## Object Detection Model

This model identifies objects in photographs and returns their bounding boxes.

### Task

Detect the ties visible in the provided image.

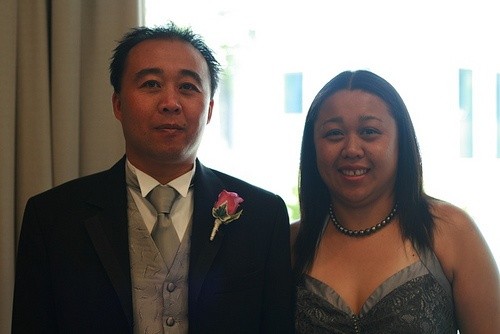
[144,185,181,272]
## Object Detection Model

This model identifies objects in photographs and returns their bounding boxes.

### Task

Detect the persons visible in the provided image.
[290,70,500,334]
[11,26,290,334]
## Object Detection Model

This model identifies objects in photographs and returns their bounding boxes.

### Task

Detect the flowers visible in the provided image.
[209,190,244,241]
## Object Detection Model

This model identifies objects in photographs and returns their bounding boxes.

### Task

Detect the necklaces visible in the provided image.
[329,200,399,237]
